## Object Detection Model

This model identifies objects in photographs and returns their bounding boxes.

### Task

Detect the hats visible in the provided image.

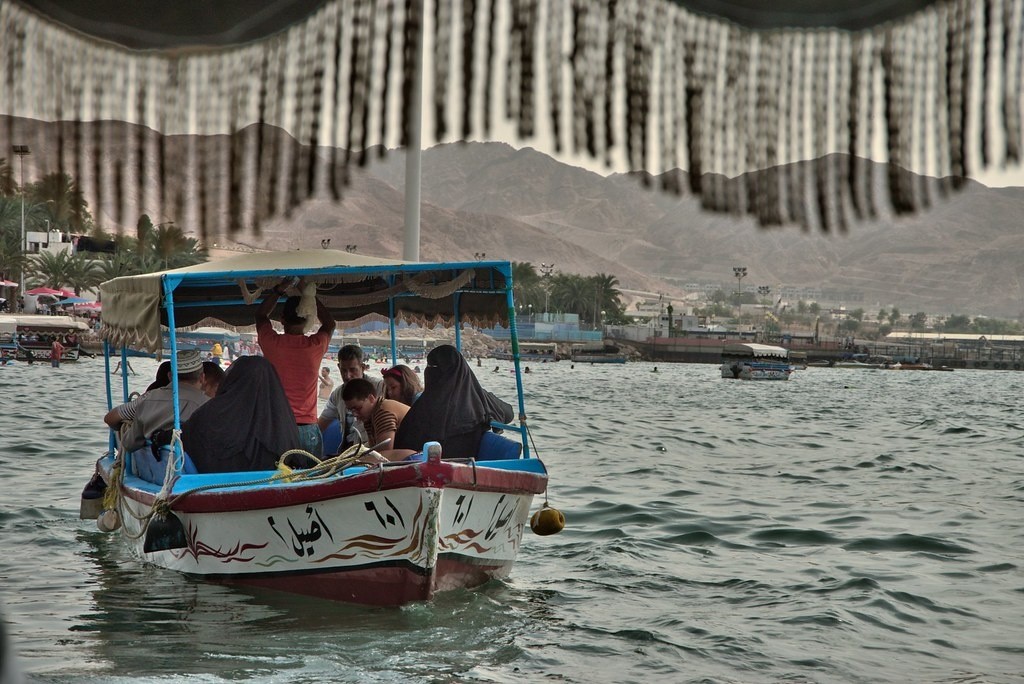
[177,348,203,373]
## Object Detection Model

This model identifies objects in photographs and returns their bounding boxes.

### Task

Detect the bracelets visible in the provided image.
[273,285,284,296]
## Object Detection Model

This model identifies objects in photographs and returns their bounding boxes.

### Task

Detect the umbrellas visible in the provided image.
[0,280,101,321]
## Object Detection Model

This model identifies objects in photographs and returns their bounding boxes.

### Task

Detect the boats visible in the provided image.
[80,246,549,614]
[788,351,808,371]
[321,335,433,360]
[81,340,127,357]
[175,327,240,365]
[571,343,626,364]
[721,343,793,381]
[491,341,558,361]
[16,321,89,362]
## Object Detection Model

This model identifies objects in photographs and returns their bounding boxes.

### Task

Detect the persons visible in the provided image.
[3,300,9,311]
[17,297,24,311]
[362,351,421,373]
[50,336,64,367]
[181,356,305,474]
[201,338,262,365]
[380,345,514,462]
[463,348,469,358]
[527,349,554,362]
[342,378,411,450]
[0,344,37,365]
[255,275,336,464]
[112,360,136,376]
[477,356,481,365]
[494,367,499,372]
[319,345,388,452]
[383,364,424,407]
[51,304,56,316]
[319,367,334,399]
[105,350,226,431]
[524,367,531,372]
